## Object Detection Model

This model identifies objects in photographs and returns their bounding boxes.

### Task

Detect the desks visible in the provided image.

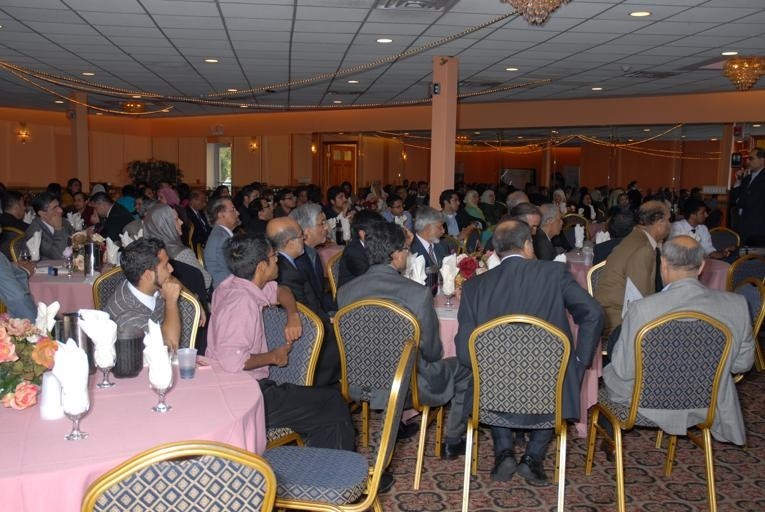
[11,255,114,316]
[8,338,268,509]
[424,286,614,426]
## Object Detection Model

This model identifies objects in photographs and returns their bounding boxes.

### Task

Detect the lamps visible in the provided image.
[723,56,765,91]
[122,102,144,113]
[501,0,570,24]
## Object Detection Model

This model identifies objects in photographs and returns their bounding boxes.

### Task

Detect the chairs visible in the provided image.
[591,311,730,512]
[139,280,208,355]
[333,306,450,454]
[270,334,422,511]
[453,309,572,512]
[250,305,321,443]
[81,436,276,512]
[92,273,149,310]
[10,202,756,260]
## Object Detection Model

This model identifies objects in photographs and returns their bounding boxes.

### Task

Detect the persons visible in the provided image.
[438,178,735,333]
[102,236,184,351]
[205,181,450,387]
[0,177,211,324]
[729,146,765,245]
[205,232,395,493]
[336,222,472,460]
[601,236,756,460]
[454,221,606,486]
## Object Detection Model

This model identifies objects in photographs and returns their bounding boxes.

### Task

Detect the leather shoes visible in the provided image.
[396,421,419,442]
[441,438,465,460]
[601,439,616,462]
[378,474,395,493]
[490,450,517,482]
[516,455,549,487]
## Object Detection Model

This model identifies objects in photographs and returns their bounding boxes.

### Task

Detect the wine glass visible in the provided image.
[441,287,456,306]
[575,242,583,256]
[61,256,74,277]
[60,386,91,440]
[146,365,175,413]
[93,343,117,389]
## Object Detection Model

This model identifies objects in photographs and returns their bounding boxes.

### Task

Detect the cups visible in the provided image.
[113,326,146,379]
[176,348,198,380]
[62,310,96,375]
[18,249,32,262]
[426,272,439,297]
[83,242,100,278]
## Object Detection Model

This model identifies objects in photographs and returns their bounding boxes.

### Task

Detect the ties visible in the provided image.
[428,244,437,264]
[656,247,663,293]
[197,212,205,247]
